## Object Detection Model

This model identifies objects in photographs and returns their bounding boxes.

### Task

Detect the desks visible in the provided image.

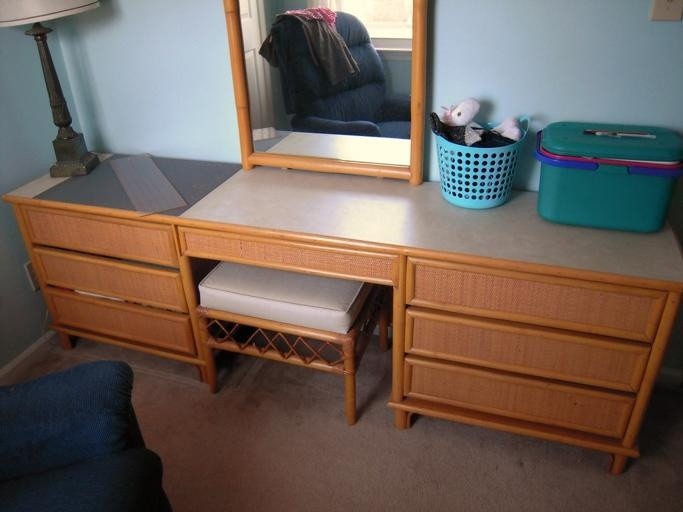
[2,151,682,476]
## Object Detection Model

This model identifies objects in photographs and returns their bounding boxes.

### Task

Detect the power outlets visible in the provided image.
[650,0,682,21]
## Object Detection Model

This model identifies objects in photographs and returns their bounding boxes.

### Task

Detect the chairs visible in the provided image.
[274,7,410,140]
[0,361,173,511]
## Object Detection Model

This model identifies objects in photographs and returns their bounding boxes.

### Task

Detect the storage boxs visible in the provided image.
[531,121,683,234]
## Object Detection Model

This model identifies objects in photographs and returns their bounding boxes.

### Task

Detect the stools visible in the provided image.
[196,262,388,426]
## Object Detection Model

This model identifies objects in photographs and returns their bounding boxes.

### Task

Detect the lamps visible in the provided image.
[1,1,102,178]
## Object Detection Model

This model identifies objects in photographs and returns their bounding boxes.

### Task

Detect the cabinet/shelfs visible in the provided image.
[397,255,681,477]
[4,197,205,392]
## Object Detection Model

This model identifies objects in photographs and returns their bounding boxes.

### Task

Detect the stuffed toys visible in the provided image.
[422,96,522,154]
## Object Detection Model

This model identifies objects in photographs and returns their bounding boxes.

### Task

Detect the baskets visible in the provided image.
[430,116,530,209]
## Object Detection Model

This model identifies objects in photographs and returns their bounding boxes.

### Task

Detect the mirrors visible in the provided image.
[224,1,428,185]
